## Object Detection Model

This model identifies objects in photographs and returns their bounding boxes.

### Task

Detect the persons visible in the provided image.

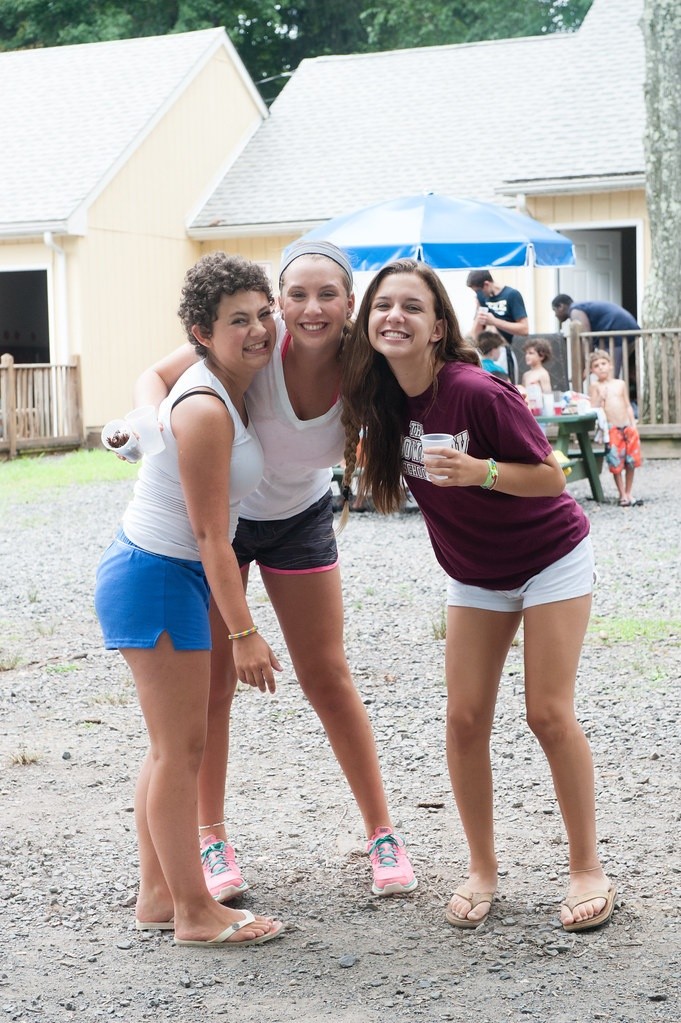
[337,257,618,927]
[521,338,552,394]
[552,294,640,379]
[116,240,418,900]
[467,270,530,345]
[95,252,285,945]
[477,331,506,373]
[588,350,643,507]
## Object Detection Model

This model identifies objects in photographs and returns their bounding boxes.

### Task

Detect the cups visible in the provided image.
[478,307,489,315]
[122,404,165,458]
[101,419,145,464]
[420,433,454,482]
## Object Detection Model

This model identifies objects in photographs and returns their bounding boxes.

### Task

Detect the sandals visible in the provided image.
[632,498,644,506]
[618,499,630,506]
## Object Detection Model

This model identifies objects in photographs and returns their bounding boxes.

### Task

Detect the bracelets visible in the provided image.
[480,458,498,491]
[228,626,258,640]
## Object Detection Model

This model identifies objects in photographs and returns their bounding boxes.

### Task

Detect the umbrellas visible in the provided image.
[282,192,577,274]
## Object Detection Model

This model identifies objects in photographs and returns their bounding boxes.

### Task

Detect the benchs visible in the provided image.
[544,446,618,501]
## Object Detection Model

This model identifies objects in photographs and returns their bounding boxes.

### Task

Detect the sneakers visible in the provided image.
[365,826,426,895]
[200,834,251,901]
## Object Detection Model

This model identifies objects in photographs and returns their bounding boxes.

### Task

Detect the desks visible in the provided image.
[535,412,605,502]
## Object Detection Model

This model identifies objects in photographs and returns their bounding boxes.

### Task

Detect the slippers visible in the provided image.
[171,909,283,947]
[562,887,625,930]
[135,914,177,931]
[445,884,498,926]
